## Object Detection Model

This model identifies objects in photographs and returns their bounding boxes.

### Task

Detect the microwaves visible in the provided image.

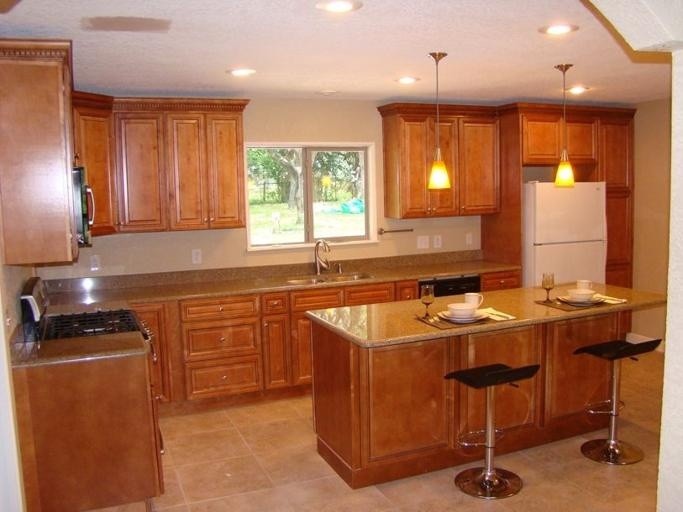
[70,165,96,248]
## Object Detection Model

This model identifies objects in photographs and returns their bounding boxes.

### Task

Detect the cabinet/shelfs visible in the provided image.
[482,269,523,291]
[181,291,292,414]
[379,103,502,219]
[520,110,598,166]
[291,280,420,397]
[600,106,636,288]
[1,38,113,266]
[112,96,250,230]
[134,302,185,417]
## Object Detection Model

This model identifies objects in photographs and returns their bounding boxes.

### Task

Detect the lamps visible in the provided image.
[554,64,576,187]
[427,52,452,189]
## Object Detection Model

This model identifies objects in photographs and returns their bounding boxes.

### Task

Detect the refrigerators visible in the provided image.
[521,180,608,288]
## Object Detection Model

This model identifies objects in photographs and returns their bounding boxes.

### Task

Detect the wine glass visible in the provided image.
[541,272,555,303]
[421,284,435,319]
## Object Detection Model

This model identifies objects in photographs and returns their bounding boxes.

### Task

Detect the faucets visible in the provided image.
[314,237,330,275]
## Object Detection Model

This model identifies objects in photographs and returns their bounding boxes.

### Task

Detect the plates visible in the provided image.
[437,310,490,324]
[557,295,607,307]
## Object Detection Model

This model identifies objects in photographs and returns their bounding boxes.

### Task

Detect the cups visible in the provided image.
[464,293,484,308]
[577,279,592,291]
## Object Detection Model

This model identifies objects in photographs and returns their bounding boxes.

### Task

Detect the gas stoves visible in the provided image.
[20,276,152,346]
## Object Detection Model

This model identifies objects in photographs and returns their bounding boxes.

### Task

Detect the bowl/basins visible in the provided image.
[568,289,595,300]
[447,303,475,316]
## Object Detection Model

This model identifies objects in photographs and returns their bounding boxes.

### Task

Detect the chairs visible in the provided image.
[445,364,540,500]
[573,335,663,464]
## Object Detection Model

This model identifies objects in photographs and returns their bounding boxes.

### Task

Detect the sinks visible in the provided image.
[285,277,333,286]
[328,272,374,283]
